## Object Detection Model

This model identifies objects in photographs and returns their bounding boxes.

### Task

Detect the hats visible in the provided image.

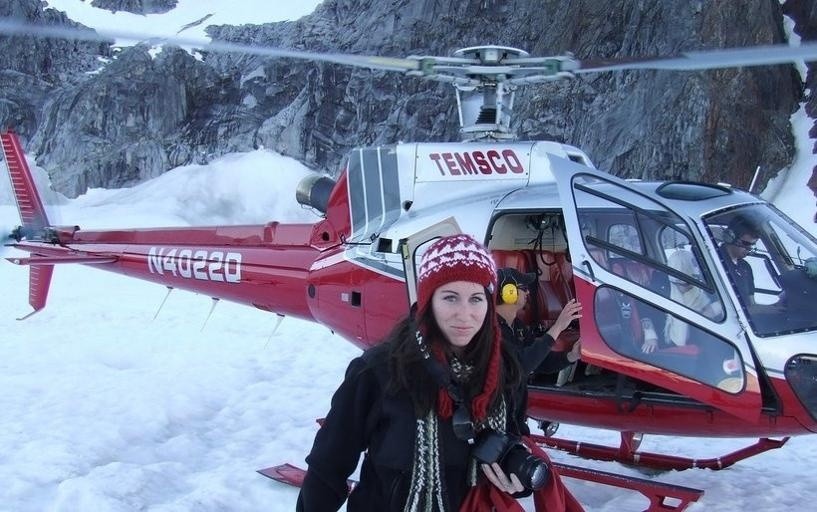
[414,233,499,310]
[497,267,537,302]
[666,248,701,284]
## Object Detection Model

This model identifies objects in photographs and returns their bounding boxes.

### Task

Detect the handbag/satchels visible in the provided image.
[461,436,585,511]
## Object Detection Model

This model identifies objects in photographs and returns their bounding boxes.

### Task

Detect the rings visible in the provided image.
[504,480,511,490]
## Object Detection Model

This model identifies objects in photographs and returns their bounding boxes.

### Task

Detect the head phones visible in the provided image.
[722,226,736,243]
[499,267,518,305]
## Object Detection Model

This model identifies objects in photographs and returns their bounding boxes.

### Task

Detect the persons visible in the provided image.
[720,214,763,306]
[494,267,584,376]
[293,233,533,511]
[634,250,715,354]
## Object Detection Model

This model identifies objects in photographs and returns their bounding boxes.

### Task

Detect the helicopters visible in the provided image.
[0,24,817,512]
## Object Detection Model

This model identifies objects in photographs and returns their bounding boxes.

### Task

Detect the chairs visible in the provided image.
[491,248,701,378]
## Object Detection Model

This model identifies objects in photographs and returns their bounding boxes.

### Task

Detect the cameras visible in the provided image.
[470,428,549,491]
